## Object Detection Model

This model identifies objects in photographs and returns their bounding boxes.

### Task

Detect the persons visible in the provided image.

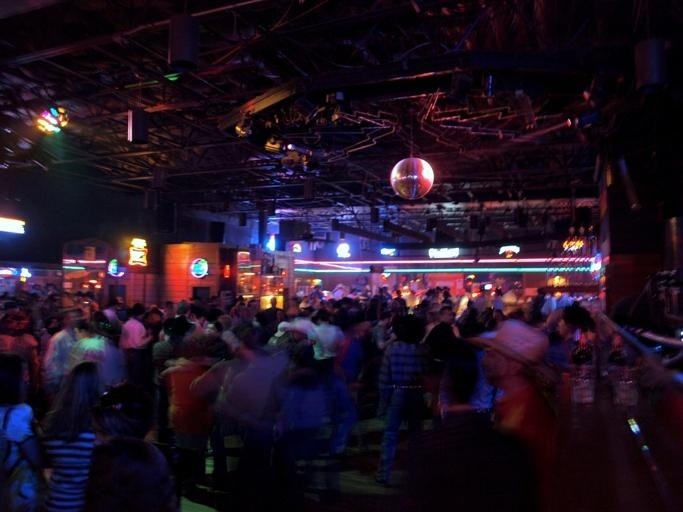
[1,271,681,512]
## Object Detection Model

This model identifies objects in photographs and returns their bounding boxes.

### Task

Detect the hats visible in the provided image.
[464,318,561,385]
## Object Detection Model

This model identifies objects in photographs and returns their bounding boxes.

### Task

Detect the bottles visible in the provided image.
[567,329,640,412]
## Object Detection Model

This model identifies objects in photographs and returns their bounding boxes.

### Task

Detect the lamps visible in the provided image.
[389,115,434,202]
